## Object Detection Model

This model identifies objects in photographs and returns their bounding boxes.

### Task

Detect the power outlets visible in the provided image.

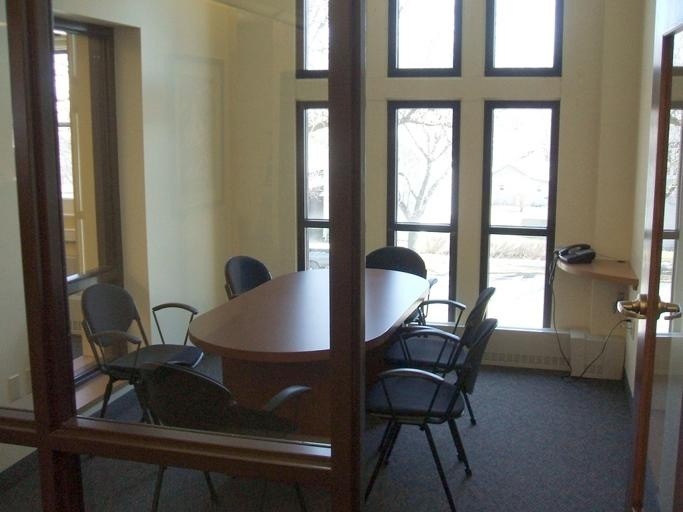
[627,318,636,341]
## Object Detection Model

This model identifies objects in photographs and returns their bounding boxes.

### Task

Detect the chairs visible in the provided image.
[364,317,496,511]
[137,361,312,512]
[80,283,203,423]
[378,288,496,461]
[224,255,273,301]
[365,245,436,324]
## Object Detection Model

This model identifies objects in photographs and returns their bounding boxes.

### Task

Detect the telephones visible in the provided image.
[559,244,596,263]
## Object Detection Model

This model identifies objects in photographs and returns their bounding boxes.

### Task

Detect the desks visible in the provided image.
[186,269,430,490]
